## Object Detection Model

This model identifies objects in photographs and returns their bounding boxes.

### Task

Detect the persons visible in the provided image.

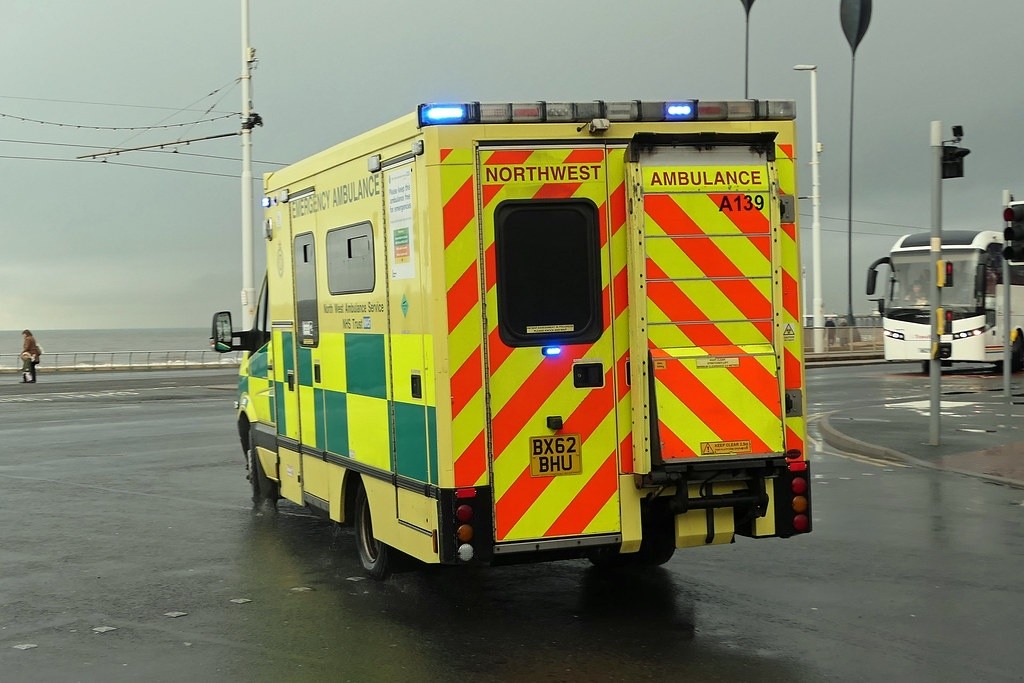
[20,330,40,383]
[903,280,927,301]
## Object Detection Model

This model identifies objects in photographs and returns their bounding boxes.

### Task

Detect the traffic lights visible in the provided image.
[1001,201,1024,265]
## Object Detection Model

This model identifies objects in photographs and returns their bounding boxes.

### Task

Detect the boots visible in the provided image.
[19,372,36,383]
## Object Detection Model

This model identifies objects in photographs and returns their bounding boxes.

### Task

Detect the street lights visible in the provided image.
[793,64,826,352]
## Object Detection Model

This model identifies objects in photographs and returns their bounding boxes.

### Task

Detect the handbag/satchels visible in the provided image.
[36,344,43,355]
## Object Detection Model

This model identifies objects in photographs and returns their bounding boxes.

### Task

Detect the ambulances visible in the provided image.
[210,99,814,579]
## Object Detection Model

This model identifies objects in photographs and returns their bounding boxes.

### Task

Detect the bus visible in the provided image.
[866,227,1024,371]
